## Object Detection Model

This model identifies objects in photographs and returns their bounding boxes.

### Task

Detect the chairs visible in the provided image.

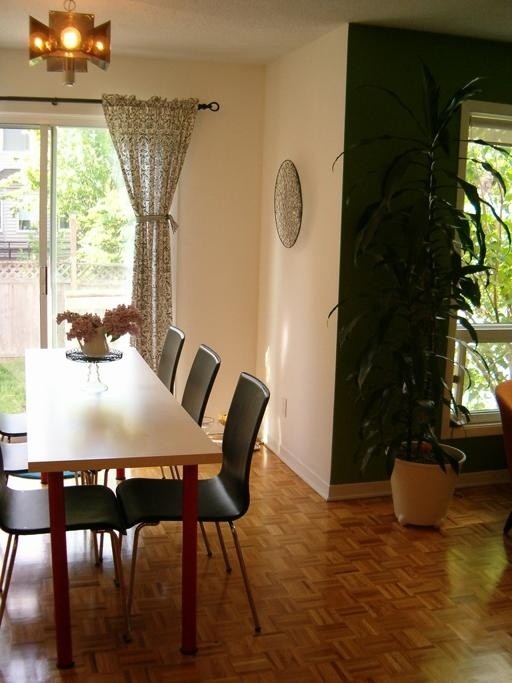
[0,441,28,486]
[157,327,187,395]
[0,443,133,643]
[1,411,27,442]
[115,372,270,635]
[99,344,222,560]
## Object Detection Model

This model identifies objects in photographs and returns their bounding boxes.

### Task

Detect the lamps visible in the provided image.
[28,2,111,84]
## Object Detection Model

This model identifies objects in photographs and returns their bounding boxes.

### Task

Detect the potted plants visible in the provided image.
[327,69,510,531]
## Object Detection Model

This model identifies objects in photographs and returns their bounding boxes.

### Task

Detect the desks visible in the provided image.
[23,344,224,668]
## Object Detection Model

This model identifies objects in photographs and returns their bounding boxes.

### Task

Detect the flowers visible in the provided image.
[56,302,147,354]
